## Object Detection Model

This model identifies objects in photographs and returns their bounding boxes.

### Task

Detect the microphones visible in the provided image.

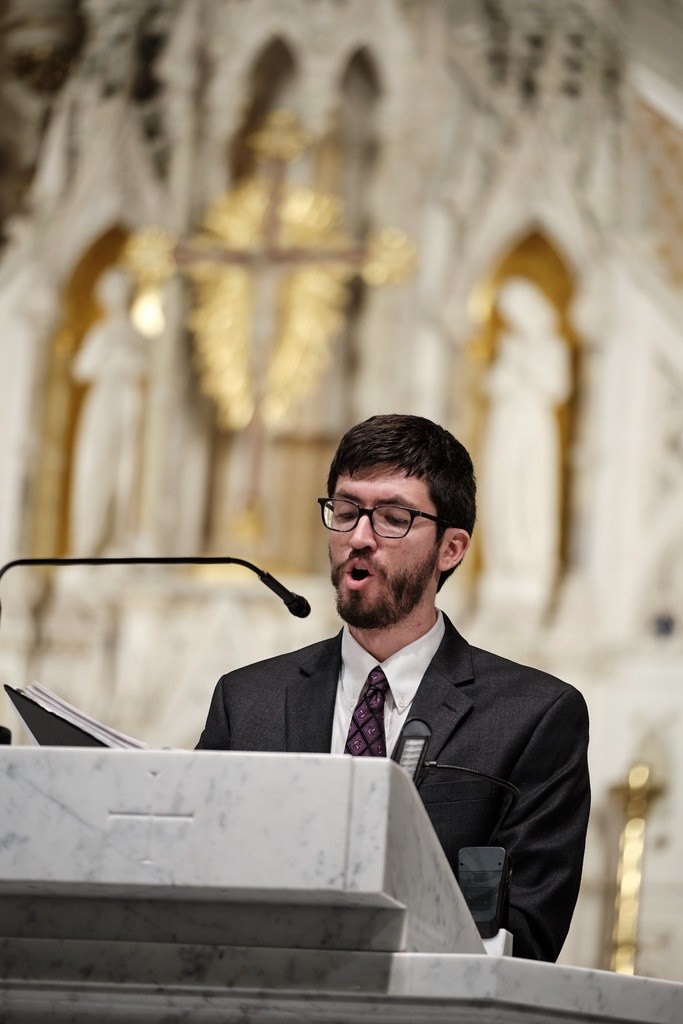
[258,569,311,619]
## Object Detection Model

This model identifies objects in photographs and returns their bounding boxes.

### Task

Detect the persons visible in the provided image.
[192,414,592,970]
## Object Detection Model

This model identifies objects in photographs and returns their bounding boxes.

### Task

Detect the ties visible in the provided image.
[343,665,389,757]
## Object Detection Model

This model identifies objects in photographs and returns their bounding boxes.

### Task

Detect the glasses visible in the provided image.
[318,496,452,538]
